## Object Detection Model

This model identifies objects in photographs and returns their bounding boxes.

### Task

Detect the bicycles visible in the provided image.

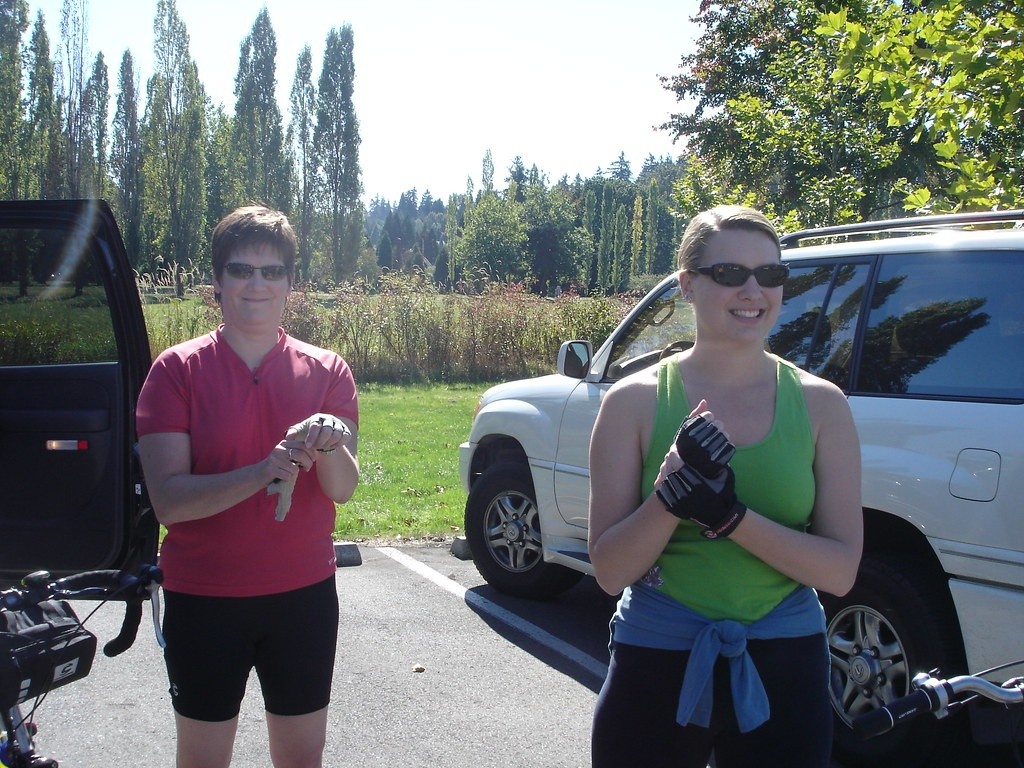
[851,658,1024,739]
[0,566,165,768]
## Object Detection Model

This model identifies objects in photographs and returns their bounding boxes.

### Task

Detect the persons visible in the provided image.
[137,206,359,768]
[588,206,864,768]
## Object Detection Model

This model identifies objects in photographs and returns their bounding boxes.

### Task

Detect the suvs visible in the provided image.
[455,201,1024,768]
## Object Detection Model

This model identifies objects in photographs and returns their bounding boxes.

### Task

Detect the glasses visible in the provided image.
[687,263,790,287]
[225,262,289,282]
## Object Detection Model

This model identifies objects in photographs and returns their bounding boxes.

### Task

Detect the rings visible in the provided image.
[289,449,293,459]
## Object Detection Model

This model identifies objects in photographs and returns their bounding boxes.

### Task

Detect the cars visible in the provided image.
[1,198,154,604]
